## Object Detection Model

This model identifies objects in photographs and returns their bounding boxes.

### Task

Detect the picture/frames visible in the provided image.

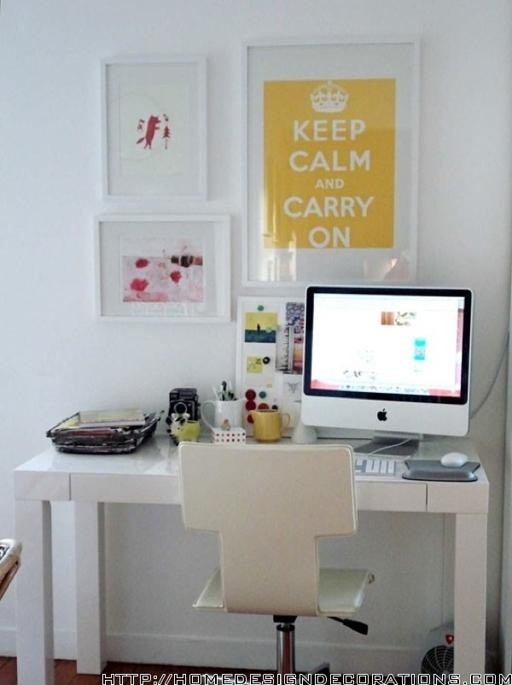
[91,32,424,327]
[11,439,490,685]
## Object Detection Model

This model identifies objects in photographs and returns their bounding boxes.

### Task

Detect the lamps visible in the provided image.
[176,440,376,685]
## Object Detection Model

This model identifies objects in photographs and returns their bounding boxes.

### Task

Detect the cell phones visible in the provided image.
[167,388,201,422]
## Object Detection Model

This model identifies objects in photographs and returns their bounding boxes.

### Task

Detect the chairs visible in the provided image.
[176,440,376,685]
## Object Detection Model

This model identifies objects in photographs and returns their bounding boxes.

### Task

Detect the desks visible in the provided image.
[11,439,490,685]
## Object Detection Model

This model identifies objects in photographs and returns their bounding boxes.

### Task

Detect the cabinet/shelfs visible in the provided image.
[291,401,318,444]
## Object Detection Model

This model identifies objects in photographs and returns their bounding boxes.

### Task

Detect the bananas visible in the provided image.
[354,457,396,478]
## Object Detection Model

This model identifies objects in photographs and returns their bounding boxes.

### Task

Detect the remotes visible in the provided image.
[441,452,470,468]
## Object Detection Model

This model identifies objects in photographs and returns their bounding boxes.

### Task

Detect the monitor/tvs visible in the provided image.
[77,408,146,429]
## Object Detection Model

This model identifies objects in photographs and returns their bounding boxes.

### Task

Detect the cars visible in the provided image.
[251,410,291,443]
[201,399,244,430]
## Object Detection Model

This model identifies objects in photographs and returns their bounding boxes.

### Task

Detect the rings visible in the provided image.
[213,381,237,401]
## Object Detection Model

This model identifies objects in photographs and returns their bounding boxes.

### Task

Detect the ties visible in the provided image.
[299,285,473,460]
